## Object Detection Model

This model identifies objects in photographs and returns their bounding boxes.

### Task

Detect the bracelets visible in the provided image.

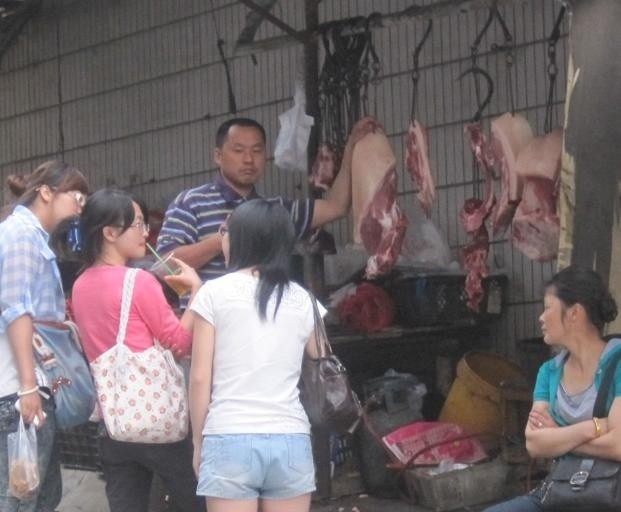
[592,417,601,438]
[17,386,39,397]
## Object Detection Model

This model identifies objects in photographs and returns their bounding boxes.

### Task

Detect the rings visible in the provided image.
[538,422,542,427]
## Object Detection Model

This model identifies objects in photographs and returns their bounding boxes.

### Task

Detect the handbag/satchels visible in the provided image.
[30,324,97,431]
[299,291,357,436]
[91,266,187,445]
[543,350,621,509]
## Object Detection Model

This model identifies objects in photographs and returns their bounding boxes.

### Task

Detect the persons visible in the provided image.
[155,116,376,312]
[188,198,328,512]
[0,161,91,512]
[49,187,203,512]
[483,266,621,512]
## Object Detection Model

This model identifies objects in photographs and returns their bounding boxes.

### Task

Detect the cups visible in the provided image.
[149,250,191,297]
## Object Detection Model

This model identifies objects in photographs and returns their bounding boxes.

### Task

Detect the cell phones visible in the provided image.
[14,399,47,428]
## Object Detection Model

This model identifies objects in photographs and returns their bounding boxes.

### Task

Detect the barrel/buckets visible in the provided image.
[438,351,528,441]
